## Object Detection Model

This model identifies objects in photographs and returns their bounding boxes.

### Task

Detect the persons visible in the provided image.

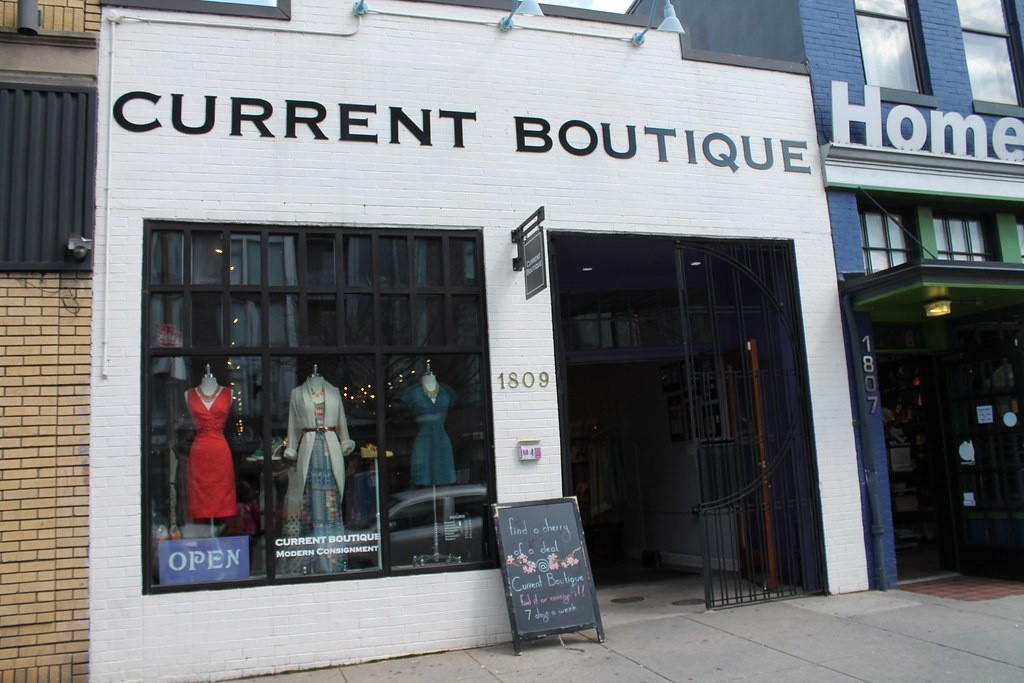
[401,360,461,485]
[284,363,356,572]
[345,457,364,529]
[179,362,237,539]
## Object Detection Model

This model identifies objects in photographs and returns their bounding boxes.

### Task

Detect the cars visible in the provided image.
[345,483,487,568]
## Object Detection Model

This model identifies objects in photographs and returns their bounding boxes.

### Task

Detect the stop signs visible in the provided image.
[155,324,181,346]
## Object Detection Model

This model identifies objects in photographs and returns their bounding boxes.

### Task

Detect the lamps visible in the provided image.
[498,0,686,47]
[924,299,951,317]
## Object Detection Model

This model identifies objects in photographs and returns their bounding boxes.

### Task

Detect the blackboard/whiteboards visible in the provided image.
[490,494,602,637]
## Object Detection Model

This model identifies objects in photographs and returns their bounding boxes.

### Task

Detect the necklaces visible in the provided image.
[423,382,440,399]
[310,387,324,397]
[197,385,220,400]
